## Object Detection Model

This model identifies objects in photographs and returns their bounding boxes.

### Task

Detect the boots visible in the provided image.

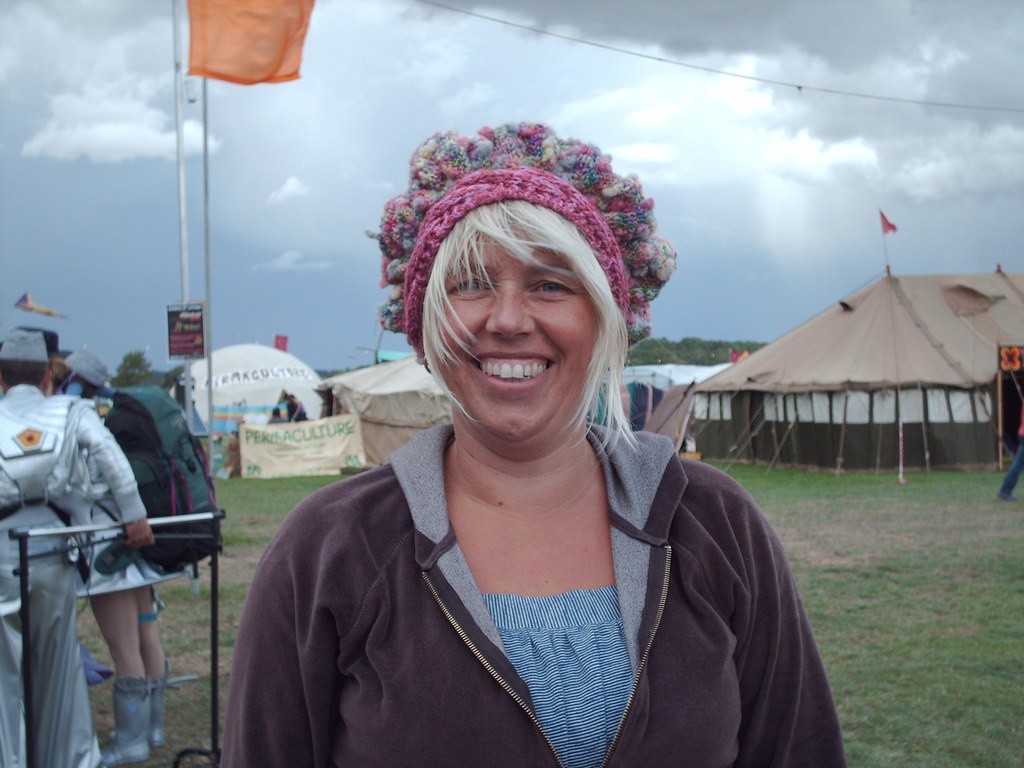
[96,676,149,768]
[109,655,169,748]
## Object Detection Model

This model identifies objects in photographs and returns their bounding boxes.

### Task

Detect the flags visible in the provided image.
[881,210,897,234]
[182,0,316,86]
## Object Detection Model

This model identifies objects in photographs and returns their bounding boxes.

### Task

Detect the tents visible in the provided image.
[169,261,1024,485]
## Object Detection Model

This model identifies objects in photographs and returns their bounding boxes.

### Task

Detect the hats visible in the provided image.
[0,330,48,362]
[0,327,72,358]
[367,122,677,365]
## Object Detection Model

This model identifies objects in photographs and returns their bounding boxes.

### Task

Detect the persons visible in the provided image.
[1,324,167,768]
[997,396,1024,504]
[222,125,844,768]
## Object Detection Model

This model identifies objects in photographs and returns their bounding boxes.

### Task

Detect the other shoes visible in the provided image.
[997,489,1016,501]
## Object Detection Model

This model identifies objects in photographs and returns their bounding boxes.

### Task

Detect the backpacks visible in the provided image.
[63,376,224,576]
